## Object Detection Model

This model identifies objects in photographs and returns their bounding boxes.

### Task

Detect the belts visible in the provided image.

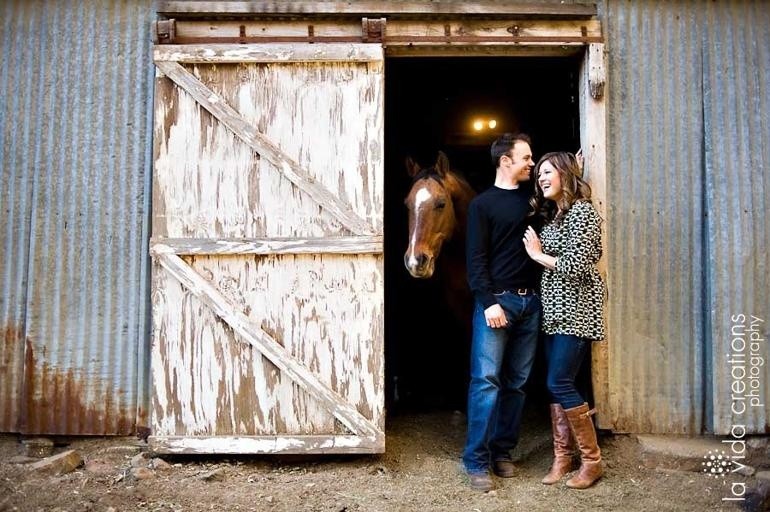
[491,288,535,296]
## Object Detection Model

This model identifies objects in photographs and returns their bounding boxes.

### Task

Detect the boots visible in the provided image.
[563,401,604,489]
[541,402,576,486]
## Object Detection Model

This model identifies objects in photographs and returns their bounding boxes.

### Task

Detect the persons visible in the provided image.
[520,146,605,491]
[461,128,586,493]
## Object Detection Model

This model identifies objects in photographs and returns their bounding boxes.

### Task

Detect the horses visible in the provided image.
[403,151,481,339]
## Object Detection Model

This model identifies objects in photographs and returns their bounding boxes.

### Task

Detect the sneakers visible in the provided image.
[468,470,494,492]
[490,457,518,478]
